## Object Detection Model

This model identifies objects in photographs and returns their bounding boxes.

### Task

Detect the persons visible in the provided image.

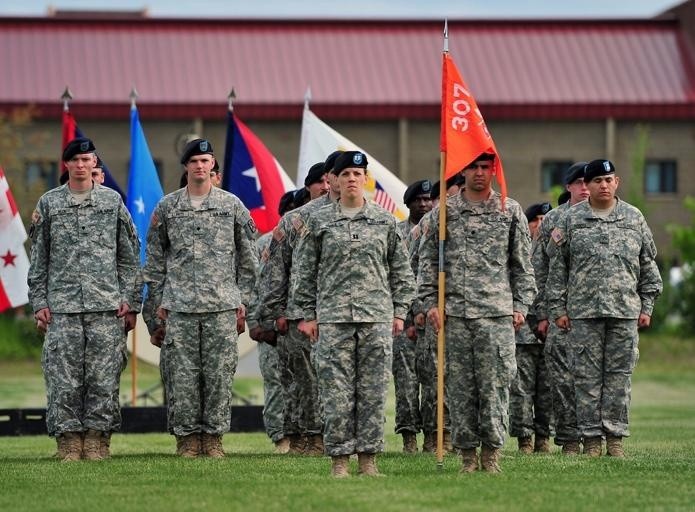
[27,137,345,463]
[414,146,541,474]
[300,149,420,480]
[397,158,663,463]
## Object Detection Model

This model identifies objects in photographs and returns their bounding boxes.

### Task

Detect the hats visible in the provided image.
[180,139,218,189]
[59,137,104,186]
[403,153,496,205]
[524,160,616,223]
[279,151,368,216]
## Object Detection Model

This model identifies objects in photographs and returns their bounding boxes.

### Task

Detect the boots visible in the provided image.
[52,431,112,463]
[176,433,225,458]
[273,435,382,479]
[402,430,503,474]
[517,435,627,458]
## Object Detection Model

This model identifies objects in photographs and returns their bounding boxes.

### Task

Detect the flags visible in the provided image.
[298,108,411,222]
[61,112,126,206]
[0,167,30,312]
[441,52,507,212]
[126,110,164,303]
[221,112,297,232]
